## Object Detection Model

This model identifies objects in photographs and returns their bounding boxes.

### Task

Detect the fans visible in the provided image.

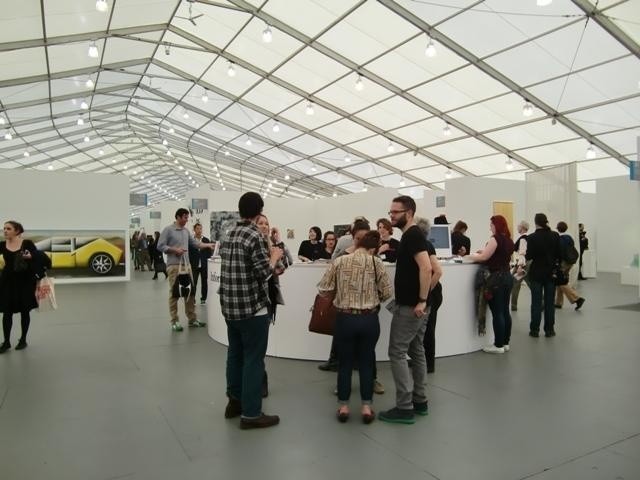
[174,3,204,26]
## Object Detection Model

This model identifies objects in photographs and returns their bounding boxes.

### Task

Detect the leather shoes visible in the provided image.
[224,403,242,418]
[529,332,539,337]
[545,331,556,337]
[0,342,11,352]
[318,363,338,372]
[15,342,27,350]
[240,414,280,429]
[555,304,561,308]
[575,298,585,310]
[362,410,375,424]
[337,408,349,422]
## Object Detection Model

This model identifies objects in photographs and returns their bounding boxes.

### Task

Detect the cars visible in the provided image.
[2,236,124,278]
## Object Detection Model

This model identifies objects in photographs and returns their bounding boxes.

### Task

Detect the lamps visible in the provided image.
[426,37,436,57]
[523,102,533,117]
[263,25,272,42]
[89,40,98,58]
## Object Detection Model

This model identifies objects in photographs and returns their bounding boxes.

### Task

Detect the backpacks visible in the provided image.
[562,236,579,264]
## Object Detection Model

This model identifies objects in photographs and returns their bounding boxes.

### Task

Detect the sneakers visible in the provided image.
[414,401,429,415]
[482,343,504,354]
[503,344,510,352]
[378,407,416,424]
[201,299,205,304]
[188,321,207,327]
[373,379,385,394]
[171,324,183,331]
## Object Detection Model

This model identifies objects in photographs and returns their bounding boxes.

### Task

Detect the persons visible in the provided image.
[218,191,289,429]
[0,221,39,354]
[331,217,384,394]
[470,215,514,354]
[298,213,470,373]
[378,195,432,425]
[524,213,562,337]
[157,208,216,332]
[506,213,589,311]
[132,221,215,305]
[317,231,392,425]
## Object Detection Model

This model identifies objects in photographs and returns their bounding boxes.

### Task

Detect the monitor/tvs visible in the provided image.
[428,224,453,259]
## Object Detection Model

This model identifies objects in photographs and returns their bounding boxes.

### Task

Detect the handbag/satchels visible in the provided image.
[172,254,196,298]
[35,273,57,313]
[309,293,339,337]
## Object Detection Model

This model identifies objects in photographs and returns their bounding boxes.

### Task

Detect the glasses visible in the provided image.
[325,238,334,241]
[388,210,407,216]
